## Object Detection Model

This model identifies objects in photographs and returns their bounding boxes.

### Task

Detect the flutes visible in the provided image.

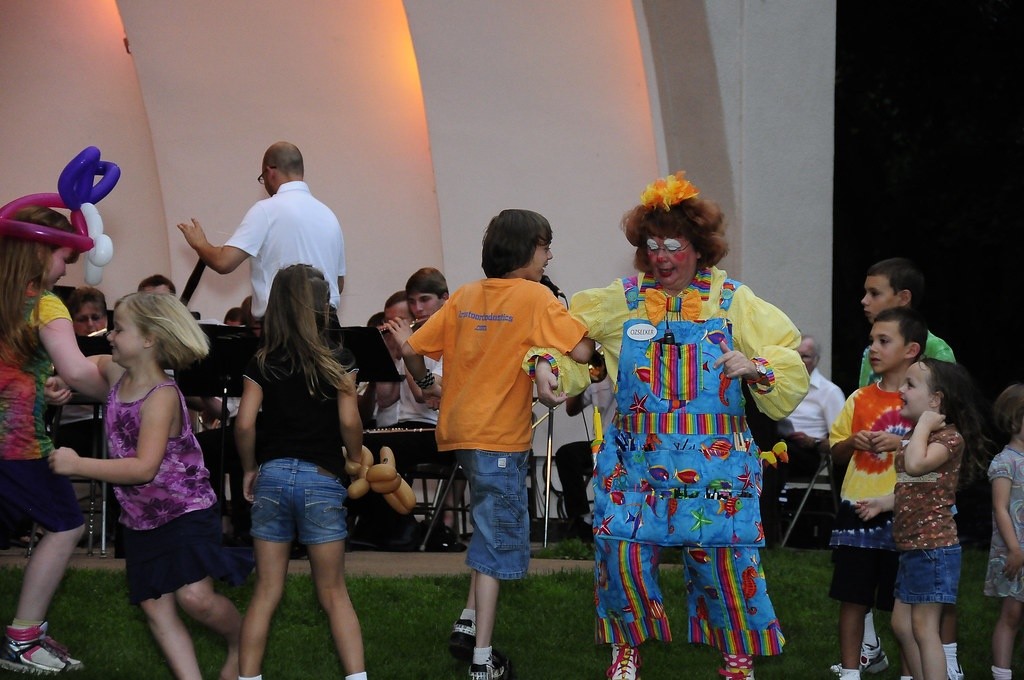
[379,314,432,335]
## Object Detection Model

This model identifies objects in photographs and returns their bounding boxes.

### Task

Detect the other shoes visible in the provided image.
[558,516,593,541]
[289,540,309,561]
[437,524,456,551]
[608,643,642,680]
[223,533,251,547]
[724,666,755,680]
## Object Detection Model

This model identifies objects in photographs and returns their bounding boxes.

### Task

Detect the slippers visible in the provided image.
[7,529,43,548]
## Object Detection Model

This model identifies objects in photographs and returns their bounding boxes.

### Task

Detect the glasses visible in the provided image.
[258,165,277,184]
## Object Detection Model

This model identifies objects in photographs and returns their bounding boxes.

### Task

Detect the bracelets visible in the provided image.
[413,369,435,389]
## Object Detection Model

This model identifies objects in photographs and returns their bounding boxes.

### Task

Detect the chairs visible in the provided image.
[26,448,852,560]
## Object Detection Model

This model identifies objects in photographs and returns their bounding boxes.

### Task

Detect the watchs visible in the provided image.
[747,360,766,384]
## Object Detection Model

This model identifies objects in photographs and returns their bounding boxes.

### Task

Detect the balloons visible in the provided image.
[341,446,416,515]
[0,147,121,286]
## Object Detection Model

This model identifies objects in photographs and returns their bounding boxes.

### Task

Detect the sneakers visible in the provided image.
[450,617,512,680]
[947,664,964,680]
[829,636,889,678]
[0,620,86,678]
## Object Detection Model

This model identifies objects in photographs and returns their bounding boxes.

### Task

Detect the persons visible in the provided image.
[43,290,244,680]
[402,209,597,680]
[237,263,366,680]
[760,257,1024,680]
[48,142,613,561]
[521,171,810,680]
[0,206,114,677]
